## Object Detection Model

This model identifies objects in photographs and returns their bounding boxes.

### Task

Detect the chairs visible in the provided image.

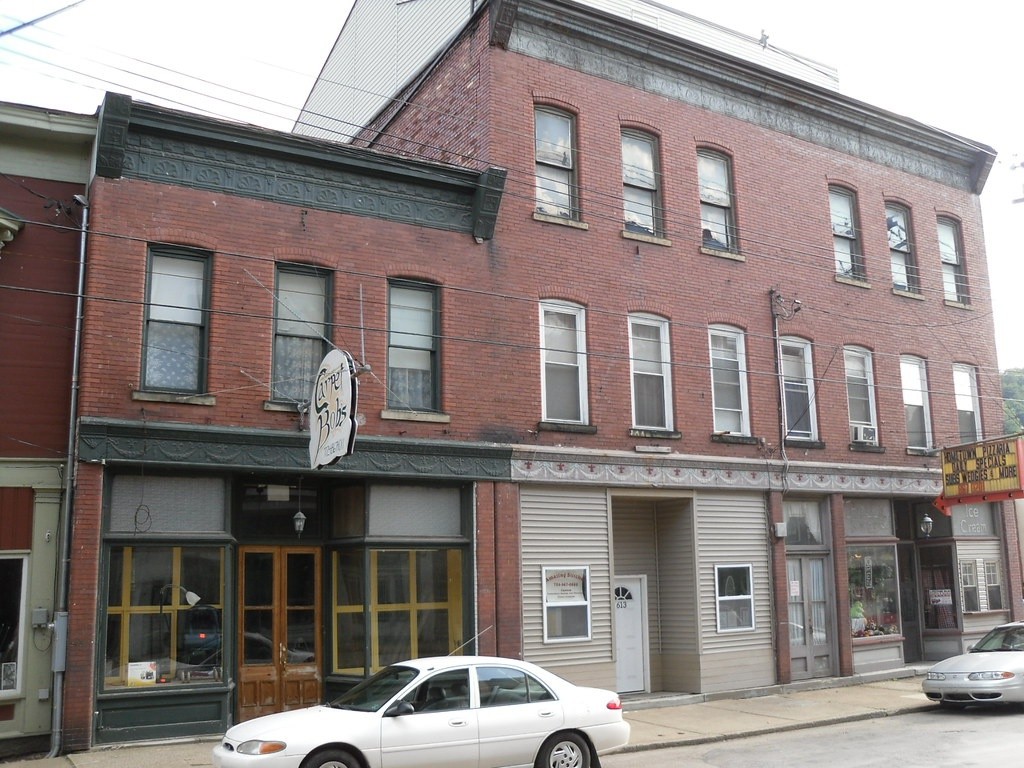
[423,686,447,708]
[451,683,468,709]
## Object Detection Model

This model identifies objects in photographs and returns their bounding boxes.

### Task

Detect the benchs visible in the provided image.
[491,687,547,703]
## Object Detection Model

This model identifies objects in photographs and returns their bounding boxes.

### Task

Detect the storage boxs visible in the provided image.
[125,662,156,686]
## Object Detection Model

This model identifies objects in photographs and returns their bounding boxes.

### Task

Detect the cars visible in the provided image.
[151,631,315,682]
[921,620,1024,709]
[780,623,825,645]
[209,655,631,768]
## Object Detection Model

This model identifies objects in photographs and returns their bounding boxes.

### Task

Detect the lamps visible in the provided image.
[920,496,934,538]
[158,584,201,683]
[292,476,307,539]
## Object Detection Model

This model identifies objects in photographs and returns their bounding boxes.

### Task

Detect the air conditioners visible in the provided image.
[851,425,876,442]
